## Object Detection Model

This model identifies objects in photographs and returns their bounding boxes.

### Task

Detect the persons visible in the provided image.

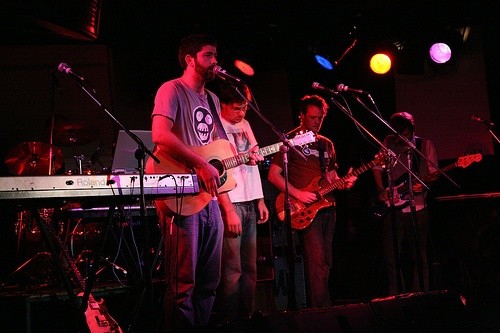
[151,34,265,329]
[372,111,439,297]
[217,80,269,325]
[268,94,358,308]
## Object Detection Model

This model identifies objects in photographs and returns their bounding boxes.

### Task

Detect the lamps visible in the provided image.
[314,20,361,70]
[368,31,404,76]
[427,21,472,66]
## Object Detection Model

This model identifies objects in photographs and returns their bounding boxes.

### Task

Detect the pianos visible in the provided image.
[1,172,201,333]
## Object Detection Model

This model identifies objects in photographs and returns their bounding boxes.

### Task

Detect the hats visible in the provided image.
[390,112,414,125]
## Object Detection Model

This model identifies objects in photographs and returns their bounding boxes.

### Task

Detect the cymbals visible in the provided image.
[14,141,64,176]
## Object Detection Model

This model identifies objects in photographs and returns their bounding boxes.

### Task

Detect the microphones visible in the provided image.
[213,65,241,83]
[57,63,84,81]
[471,115,494,126]
[337,83,364,94]
[312,82,339,95]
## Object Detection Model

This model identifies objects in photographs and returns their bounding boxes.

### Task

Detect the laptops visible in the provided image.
[111,130,154,175]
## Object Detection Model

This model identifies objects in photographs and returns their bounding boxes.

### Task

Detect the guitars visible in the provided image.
[381,153,482,207]
[275,152,392,230]
[144,130,318,217]
[53,232,123,333]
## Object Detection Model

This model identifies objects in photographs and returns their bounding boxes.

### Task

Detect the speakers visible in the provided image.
[294,302,375,333]
[371,288,465,333]
